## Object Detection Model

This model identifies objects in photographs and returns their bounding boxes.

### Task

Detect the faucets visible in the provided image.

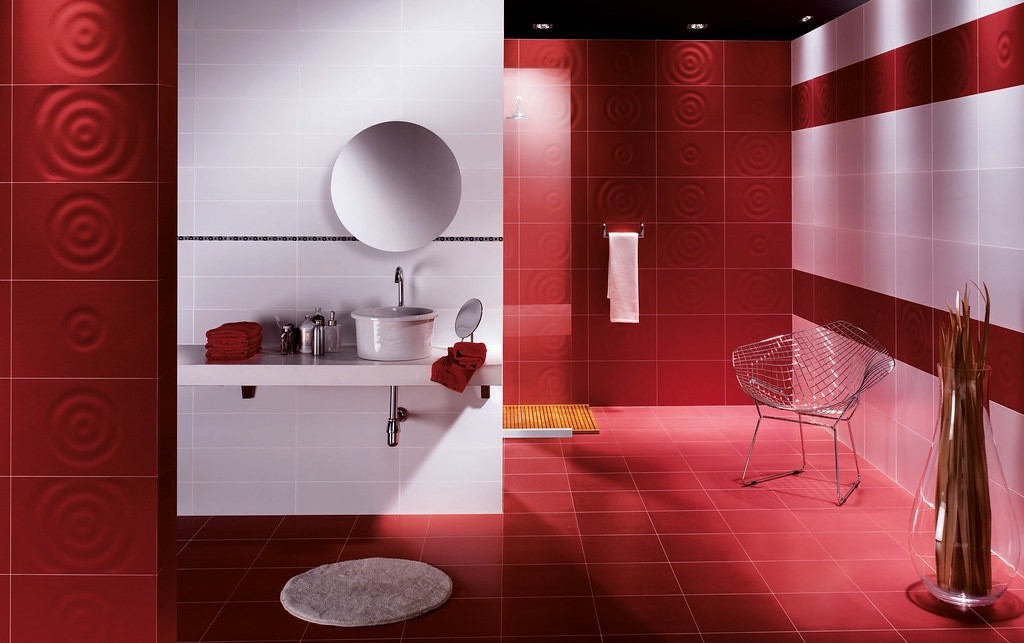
[393,265,405,307]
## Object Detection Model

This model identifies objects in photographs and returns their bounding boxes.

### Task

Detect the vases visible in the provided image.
[907,362,1021,608]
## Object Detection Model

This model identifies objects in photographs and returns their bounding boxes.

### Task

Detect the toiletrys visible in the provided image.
[280,307,342,356]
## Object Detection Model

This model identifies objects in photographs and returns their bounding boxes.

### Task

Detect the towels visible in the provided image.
[606,232,640,324]
[430,341,487,394]
[204,321,263,360]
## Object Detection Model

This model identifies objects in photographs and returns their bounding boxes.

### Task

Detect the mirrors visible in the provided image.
[454,297,484,342]
[329,121,463,252]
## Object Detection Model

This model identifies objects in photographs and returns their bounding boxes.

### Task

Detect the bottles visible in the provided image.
[297,315,315,353]
[311,307,325,325]
[280,325,295,355]
[312,320,325,356]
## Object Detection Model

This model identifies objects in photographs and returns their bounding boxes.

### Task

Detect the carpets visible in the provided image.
[281,556,453,625]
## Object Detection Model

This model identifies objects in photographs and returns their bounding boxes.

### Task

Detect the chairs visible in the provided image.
[732,319,896,506]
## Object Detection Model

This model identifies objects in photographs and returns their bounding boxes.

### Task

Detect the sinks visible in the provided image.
[350,305,439,361]
